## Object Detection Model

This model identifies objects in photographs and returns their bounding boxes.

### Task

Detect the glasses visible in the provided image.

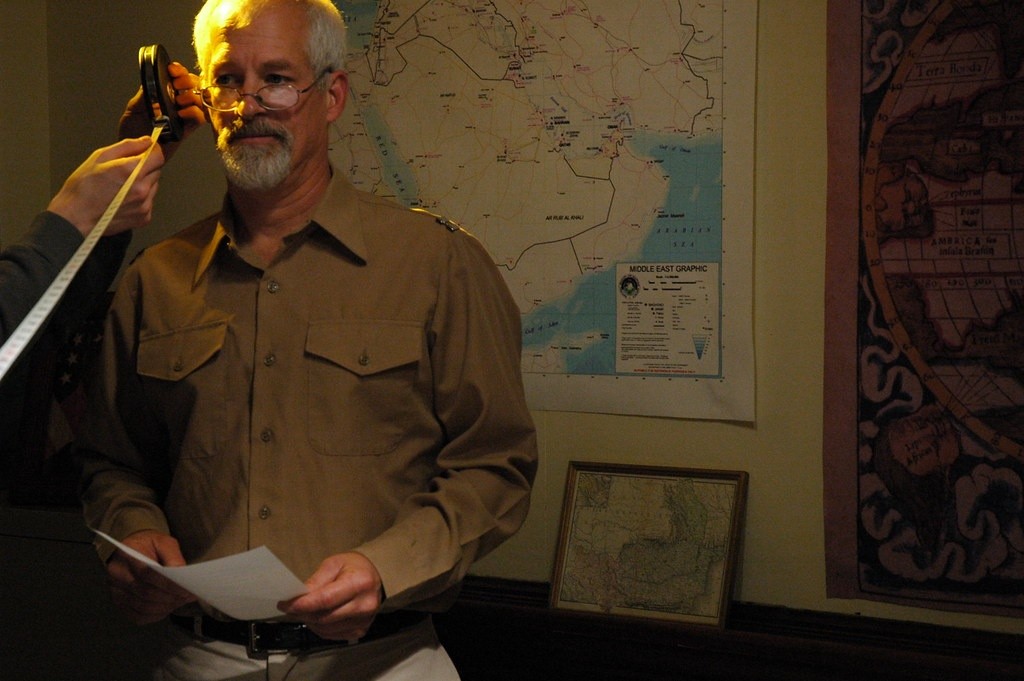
[193,63,333,111]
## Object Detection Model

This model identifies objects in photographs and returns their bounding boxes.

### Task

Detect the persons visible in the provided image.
[70,0,539,681]
[0,62,209,508]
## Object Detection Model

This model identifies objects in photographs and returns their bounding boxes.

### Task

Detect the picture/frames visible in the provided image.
[546,461,749,631]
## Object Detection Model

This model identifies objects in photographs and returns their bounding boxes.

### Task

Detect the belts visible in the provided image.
[169,610,426,652]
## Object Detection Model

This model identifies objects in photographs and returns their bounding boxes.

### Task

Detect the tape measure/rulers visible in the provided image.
[0,44,176,381]
